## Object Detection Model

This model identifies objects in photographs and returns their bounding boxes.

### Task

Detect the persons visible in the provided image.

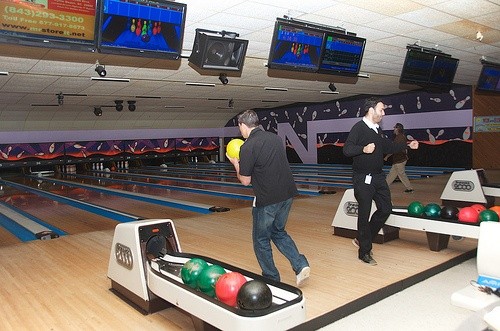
[385,123,415,193]
[226,109,311,288]
[343,97,419,266]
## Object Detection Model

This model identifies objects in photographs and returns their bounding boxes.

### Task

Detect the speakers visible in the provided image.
[188,29,249,72]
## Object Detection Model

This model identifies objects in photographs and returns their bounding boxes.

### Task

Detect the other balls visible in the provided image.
[226,139,246,161]
[180,258,273,309]
[408,202,500,226]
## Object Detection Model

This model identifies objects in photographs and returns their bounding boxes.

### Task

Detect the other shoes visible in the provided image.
[352,238,373,256]
[403,189,414,193]
[359,256,377,266]
[297,266,310,288]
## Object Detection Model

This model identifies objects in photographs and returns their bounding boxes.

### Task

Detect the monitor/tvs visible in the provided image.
[399,50,461,88]
[268,21,366,77]
[477,66,500,92]
[94,0,187,60]
